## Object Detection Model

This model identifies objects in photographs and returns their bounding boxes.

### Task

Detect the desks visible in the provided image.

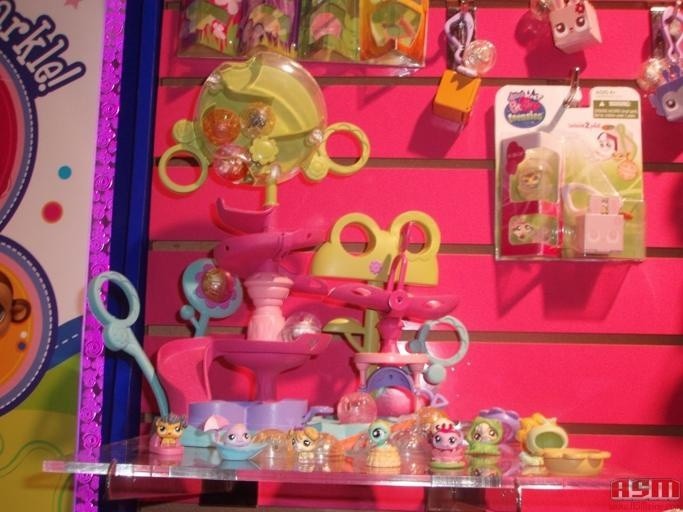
[40,429,630,510]
